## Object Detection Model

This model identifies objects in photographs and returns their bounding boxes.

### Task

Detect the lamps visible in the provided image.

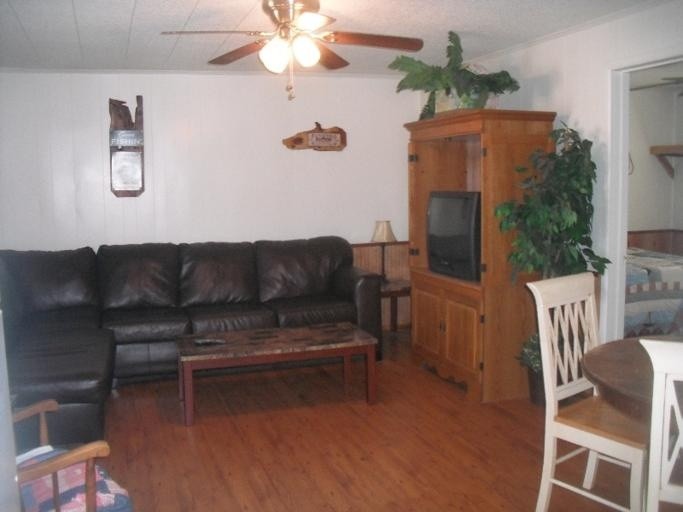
[371,221,399,284]
[258,24,321,74]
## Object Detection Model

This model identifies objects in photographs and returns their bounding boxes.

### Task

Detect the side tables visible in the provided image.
[381,276,411,343]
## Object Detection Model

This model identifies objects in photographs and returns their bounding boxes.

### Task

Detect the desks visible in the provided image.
[581,332,683,423]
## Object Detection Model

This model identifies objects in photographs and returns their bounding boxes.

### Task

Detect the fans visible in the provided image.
[161,1,425,76]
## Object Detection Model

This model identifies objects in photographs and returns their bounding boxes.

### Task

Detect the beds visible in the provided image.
[626,245,682,335]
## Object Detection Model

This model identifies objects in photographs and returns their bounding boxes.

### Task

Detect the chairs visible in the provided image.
[11,397,132,512]
[525,271,647,511]
[638,338,683,512]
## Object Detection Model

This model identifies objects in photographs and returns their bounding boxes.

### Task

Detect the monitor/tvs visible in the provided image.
[425,190,481,283]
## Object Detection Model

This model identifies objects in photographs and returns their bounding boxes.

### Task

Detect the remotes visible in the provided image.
[192,337,226,345]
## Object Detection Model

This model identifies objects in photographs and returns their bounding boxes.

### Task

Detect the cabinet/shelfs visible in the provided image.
[404,110,558,401]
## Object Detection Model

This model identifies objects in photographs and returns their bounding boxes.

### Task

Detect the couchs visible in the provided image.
[8,326,117,448]
[105,265,384,387]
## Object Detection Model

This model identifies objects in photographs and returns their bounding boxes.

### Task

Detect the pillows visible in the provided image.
[1,245,100,310]
[178,240,258,306]
[257,235,354,303]
[100,244,179,312]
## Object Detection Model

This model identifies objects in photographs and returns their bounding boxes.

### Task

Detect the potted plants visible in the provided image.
[494,125,612,407]
